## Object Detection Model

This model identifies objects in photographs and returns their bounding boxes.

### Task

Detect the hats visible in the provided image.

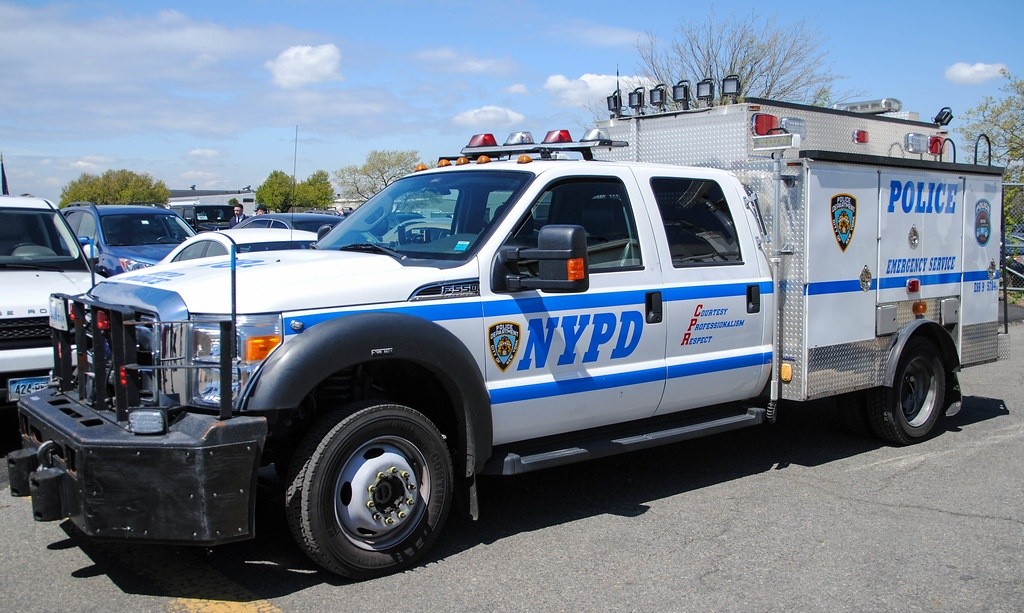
[254,206,267,212]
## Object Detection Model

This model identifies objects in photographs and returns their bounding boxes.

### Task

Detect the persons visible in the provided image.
[255,206,267,216]
[228,204,248,229]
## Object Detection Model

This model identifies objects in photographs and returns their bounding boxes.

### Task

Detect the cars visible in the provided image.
[227,212,384,252]
[381,216,481,249]
[154,228,321,268]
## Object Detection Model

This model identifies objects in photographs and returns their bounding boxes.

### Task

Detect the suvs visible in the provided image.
[62,201,202,280]
[0,194,120,412]
[168,203,253,235]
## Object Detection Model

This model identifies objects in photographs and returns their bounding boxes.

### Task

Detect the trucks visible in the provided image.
[7,74,1007,580]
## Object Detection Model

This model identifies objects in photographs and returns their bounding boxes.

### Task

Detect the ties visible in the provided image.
[238,216,239,224]
